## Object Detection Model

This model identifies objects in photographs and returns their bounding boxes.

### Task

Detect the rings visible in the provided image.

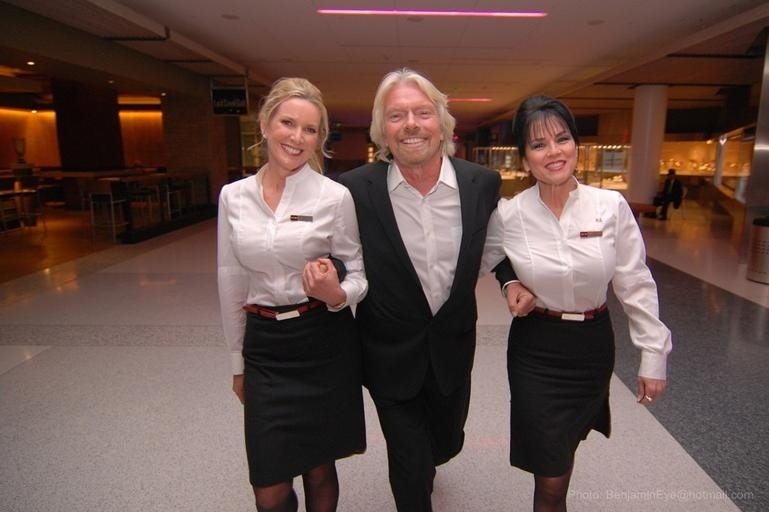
[645,395,654,403]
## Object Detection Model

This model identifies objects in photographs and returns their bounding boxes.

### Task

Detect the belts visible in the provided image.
[244,297,332,322]
[528,300,613,323]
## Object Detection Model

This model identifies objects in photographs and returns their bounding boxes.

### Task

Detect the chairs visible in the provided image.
[89,167,185,238]
[669,186,688,220]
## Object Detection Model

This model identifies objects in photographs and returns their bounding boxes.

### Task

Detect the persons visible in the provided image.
[657,168,683,221]
[476,94,674,509]
[215,74,369,512]
[319,66,537,510]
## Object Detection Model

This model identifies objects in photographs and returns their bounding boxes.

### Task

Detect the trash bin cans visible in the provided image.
[746,217,769,285]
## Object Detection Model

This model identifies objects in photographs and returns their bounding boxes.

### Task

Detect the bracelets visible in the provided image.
[329,301,346,309]
